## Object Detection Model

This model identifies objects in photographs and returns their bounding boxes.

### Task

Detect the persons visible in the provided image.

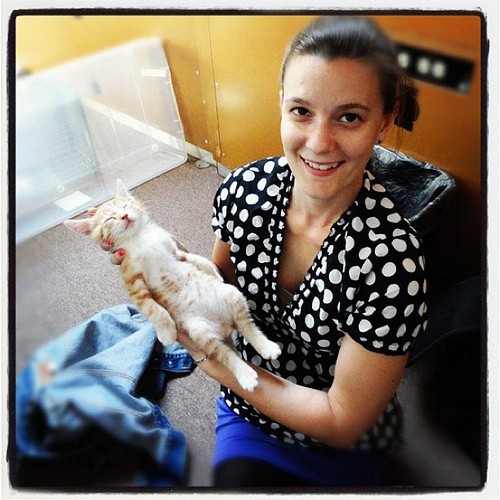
[86,15,427,490]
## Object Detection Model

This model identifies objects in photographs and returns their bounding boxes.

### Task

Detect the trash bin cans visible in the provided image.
[364,146,461,252]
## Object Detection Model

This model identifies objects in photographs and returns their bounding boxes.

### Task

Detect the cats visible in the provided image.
[62,178,282,392]
